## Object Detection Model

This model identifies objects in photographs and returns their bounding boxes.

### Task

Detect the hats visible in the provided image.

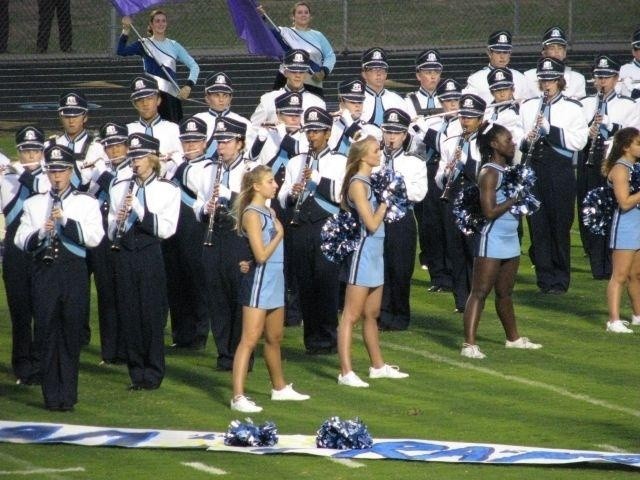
[415,50,444,70]
[337,46,410,134]
[14,90,88,168]
[591,54,621,78]
[436,78,462,101]
[274,48,334,132]
[629,29,640,50]
[540,25,568,47]
[98,74,160,159]
[489,29,514,51]
[457,93,486,119]
[487,67,514,91]
[535,56,566,80]
[177,70,247,142]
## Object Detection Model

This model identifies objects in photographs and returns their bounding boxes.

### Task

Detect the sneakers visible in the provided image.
[369,363,409,380]
[461,343,487,359]
[605,320,634,334]
[631,314,640,325]
[505,336,542,349]
[338,371,370,388]
[230,396,262,413]
[271,382,311,401]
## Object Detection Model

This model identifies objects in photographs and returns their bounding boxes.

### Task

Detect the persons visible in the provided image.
[248,28,430,352]
[228,165,311,410]
[34,0,77,53]
[401,28,639,311]
[461,121,542,358]
[601,127,640,334]
[1,77,270,412]
[1,0,13,48]
[257,3,338,101]
[335,131,410,387]
[117,11,202,124]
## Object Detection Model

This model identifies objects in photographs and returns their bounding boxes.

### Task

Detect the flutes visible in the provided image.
[586,87,605,167]
[383,141,392,167]
[522,90,548,166]
[44,182,59,265]
[290,140,313,225]
[112,165,139,251]
[259,98,520,130]
[439,125,469,203]
[204,154,223,248]
[4,148,202,167]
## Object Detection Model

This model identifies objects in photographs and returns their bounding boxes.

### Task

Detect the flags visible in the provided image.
[110,1,167,18]
[228,2,287,61]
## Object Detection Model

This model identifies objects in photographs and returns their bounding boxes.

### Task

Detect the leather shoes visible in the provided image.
[284,321,330,355]
[453,307,465,314]
[104,357,159,392]
[427,284,439,293]
[437,286,452,293]
[548,285,567,296]
[535,287,550,296]
[376,319,399,330]
[20,377,73,413]
[168,341,231,372]
